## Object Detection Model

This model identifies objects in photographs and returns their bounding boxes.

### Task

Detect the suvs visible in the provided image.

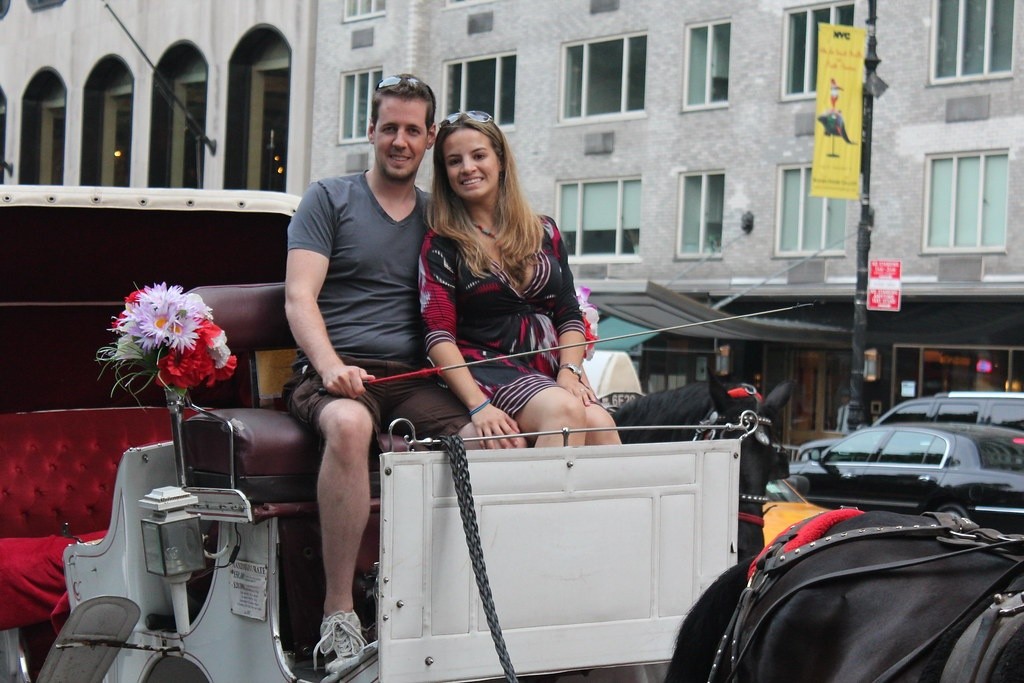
[796,391,1024,461]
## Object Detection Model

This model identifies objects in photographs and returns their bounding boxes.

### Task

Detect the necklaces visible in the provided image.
[471,222,496,239]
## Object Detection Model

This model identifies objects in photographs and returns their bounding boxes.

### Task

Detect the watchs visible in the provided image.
[558,363,582,379]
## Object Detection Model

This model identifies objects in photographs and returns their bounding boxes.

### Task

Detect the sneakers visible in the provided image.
[312,610,368,673]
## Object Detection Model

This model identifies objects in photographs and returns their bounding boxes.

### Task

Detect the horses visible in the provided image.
[612,351,794,565]
[665,510,1024,682]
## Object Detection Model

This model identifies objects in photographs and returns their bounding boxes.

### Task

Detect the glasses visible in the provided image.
[438,110,494,129]
[375,76,424,94]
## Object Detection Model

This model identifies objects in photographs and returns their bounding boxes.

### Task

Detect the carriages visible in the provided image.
[0,179,1024,683]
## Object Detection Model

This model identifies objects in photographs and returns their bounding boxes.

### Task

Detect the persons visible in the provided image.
[284,74,526,674]
[419,111,622,451]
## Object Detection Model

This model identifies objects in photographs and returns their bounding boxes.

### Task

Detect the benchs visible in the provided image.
[1,407,215,631]
[178,282,437,525]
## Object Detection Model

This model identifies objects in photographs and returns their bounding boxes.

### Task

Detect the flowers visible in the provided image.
[95,281,237,412]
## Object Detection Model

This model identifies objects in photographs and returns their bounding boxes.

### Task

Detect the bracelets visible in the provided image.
[469,399,491,416]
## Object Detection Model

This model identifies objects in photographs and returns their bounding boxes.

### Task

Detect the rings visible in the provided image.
[580,389,585,392]
[585,388,587,391]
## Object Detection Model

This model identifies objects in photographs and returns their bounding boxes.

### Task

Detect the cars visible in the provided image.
[789,423,1024,540]
